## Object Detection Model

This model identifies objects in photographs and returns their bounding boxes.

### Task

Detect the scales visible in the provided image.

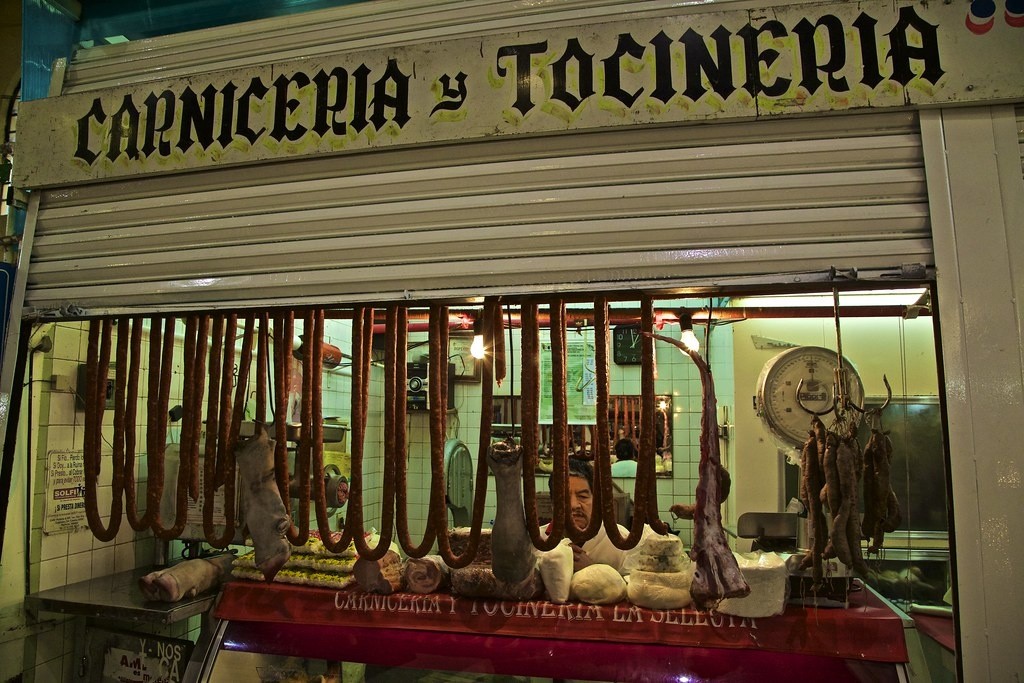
[753,344,866,610]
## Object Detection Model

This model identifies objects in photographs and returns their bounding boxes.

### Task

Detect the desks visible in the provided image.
[215,546,910,682]
[22,558,214,683]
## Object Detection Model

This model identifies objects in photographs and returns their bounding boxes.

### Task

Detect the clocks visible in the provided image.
[612,324,644,366]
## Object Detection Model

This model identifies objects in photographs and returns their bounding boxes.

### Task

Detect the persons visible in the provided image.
[607,439,637,477]
[535,459,631,567]
